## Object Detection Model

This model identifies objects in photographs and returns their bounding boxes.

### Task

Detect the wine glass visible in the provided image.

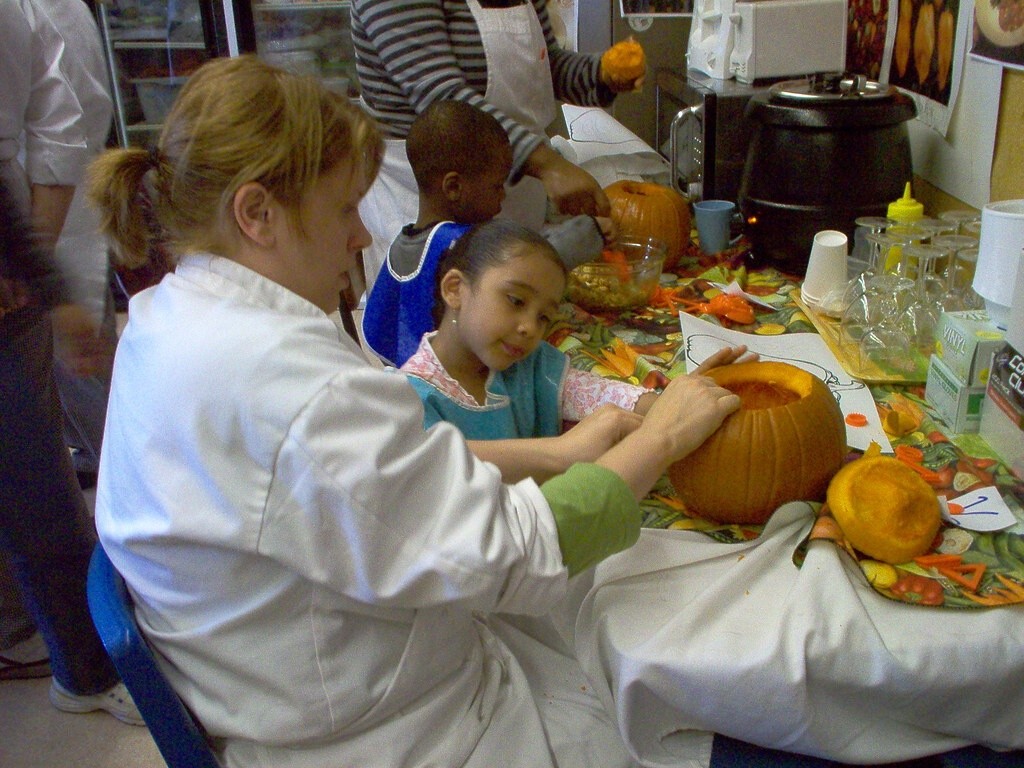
[839,210,983,377]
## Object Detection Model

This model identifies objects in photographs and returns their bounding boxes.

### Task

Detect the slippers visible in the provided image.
[0,654,49,679]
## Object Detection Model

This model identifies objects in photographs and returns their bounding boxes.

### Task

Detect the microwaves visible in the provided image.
[655,68,796,211]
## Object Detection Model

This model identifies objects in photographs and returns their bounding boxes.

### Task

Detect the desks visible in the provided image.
[544,257,1024,768]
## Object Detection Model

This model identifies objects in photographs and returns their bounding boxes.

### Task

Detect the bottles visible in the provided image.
[886,180,924,280]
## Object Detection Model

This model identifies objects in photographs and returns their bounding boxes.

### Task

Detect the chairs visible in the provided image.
[87,541,221,768]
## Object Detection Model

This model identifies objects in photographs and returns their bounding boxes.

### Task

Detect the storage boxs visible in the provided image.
[926,310,1007,434]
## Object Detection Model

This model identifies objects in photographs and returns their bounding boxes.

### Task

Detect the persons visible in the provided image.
[0,0,759,768]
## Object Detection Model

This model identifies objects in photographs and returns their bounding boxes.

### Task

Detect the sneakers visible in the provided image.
[48,675,146,725]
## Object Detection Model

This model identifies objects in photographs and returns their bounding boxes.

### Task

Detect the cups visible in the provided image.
[694,201,736,256]
[801,230,849,307]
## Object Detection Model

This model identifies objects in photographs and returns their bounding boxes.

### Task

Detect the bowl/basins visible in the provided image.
[565,235,671,311]
